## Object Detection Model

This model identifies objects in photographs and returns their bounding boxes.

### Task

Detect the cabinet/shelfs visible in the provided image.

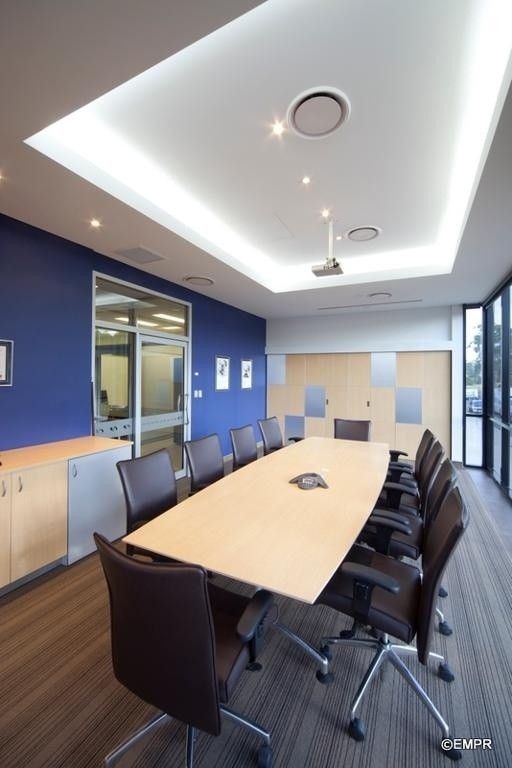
[57,445,133,566]
[1,463,68,588]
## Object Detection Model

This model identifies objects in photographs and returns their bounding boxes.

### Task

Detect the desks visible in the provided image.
[122,433,400,607]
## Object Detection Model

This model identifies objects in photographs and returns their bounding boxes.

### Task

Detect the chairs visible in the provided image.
[256,416,285,455]
[182,431,226,494]
[228,422,257,469]
[334,416,370,445]
[385,428,436,476]
[388,440,446,491]
[115,447,184,555]
[292,489,485,762]
[84,529,275,768]
[376,457,463,600]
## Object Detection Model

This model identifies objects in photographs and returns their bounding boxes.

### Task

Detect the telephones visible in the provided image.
[289,472,329,490]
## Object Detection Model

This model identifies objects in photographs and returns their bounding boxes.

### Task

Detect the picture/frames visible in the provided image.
[0,341,12,389]
[238,359,253,390]
[213,354,231,393]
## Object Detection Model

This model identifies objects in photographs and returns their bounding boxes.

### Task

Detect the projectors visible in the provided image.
[312,258,344,277]
